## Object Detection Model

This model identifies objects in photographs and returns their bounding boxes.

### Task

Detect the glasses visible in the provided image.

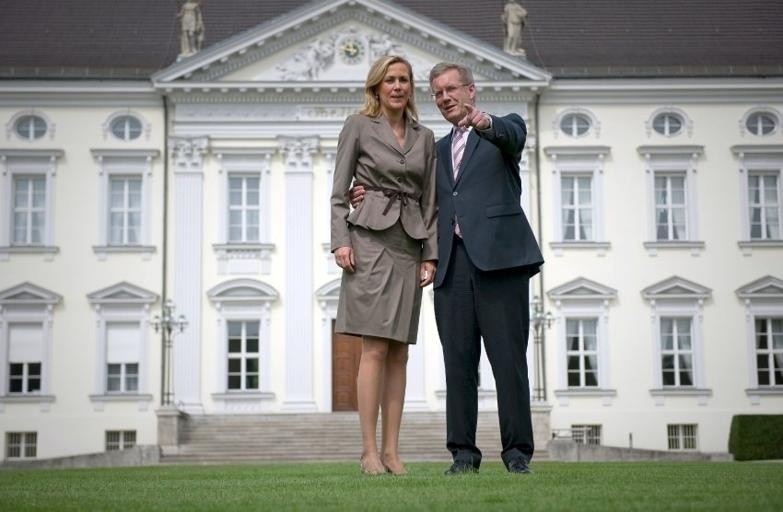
[430,82,472,100]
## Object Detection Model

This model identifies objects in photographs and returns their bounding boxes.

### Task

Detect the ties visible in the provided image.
[451,129,470,240]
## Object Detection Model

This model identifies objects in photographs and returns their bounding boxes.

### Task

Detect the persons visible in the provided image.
[348,63,544,477]
[500,1,527,54]
[176,1,203,55]
[328,54,437,476]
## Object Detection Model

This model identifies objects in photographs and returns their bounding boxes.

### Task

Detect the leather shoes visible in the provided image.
[383,456,406,476]
[359,460,388,475]
[509,460,531,474]
[444,461,477,476]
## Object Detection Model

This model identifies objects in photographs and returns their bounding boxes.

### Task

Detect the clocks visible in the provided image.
[336,35,368,68]
